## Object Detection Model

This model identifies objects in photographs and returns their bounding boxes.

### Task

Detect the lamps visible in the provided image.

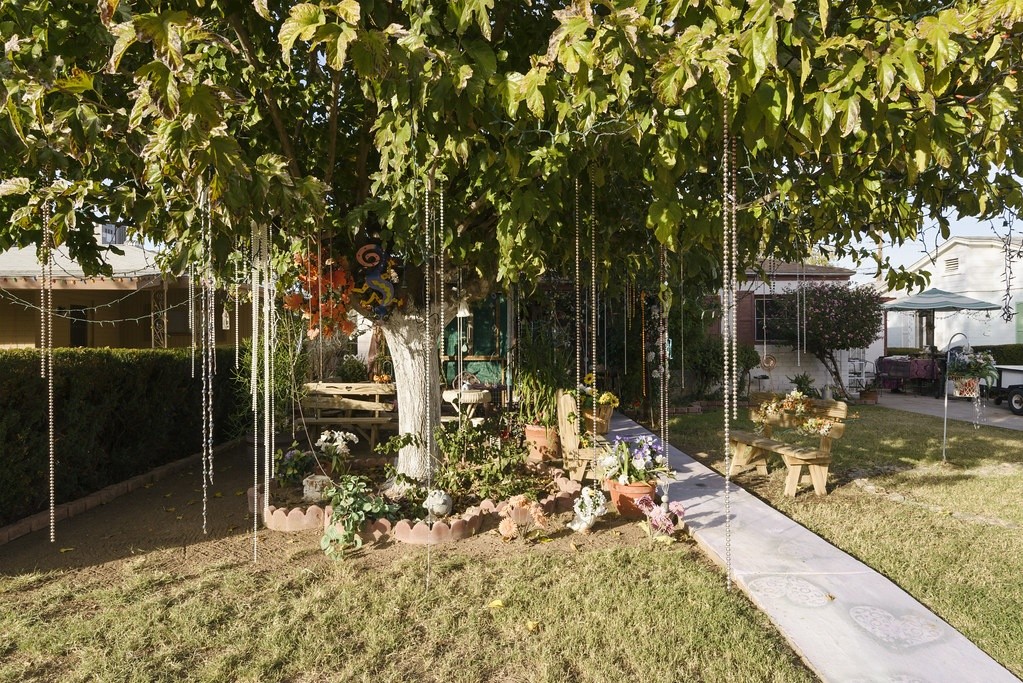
[919,310,929,317]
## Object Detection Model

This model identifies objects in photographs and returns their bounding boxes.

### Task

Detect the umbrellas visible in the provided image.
[879,288,1002,379]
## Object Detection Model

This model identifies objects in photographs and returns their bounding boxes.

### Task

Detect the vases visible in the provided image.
[583,405,613,436]
[605,479,656,519]
[950,376,981,398]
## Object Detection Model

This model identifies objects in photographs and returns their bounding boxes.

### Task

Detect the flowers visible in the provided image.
[946,346,999,387]
[562,371,678,490]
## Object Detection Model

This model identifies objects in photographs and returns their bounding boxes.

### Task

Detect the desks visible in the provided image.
[443,389,491,421]
[883,355,940,398]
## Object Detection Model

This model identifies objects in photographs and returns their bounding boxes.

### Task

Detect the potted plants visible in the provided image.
[857,376,880,404]
[498,322,587,460]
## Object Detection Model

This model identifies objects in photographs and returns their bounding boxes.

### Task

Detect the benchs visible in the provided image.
[556,389,612,484]
[296,383,395,453]
[716,388,848,500]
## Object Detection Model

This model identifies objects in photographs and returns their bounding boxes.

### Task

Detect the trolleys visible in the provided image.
[978,364,1023,415]
